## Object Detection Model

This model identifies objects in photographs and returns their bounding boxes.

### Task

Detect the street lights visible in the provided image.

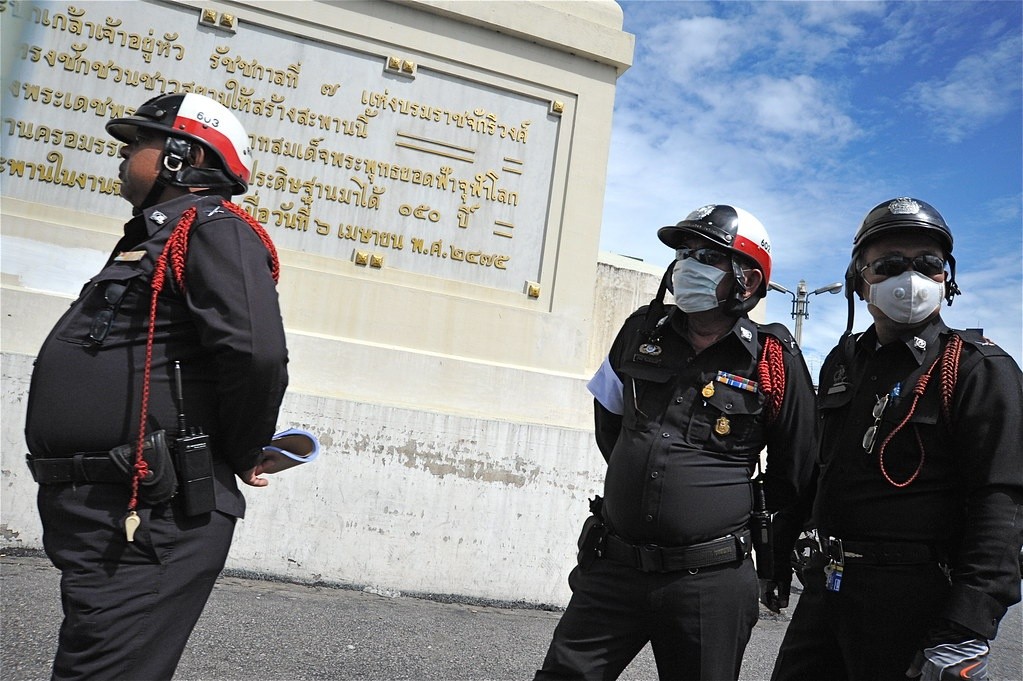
[766,278,845,350]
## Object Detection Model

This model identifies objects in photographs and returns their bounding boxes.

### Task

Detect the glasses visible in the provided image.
[856,254,944,276]
[675,244,732,266]
[862,393,889,455]
[81,279,133,352]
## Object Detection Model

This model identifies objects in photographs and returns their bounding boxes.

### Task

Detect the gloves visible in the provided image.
[905,619,991,681]
[756,538,792,614]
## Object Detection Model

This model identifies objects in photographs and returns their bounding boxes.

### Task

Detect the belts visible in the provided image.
[24,453,129,485]
[818,533,962,566]
[594,526,752,574]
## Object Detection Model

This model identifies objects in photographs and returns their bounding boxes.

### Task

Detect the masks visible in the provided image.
[671,257,752,314]
[858,273,946,324]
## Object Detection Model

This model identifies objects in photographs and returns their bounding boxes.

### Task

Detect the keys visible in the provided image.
[824,559,833,588]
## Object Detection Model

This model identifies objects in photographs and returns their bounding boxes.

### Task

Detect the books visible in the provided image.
[257,427,319,474]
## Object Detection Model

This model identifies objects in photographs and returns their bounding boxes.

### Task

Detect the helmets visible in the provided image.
[657,205,771,298]
[105,92,255,196]
[852,197,952,264]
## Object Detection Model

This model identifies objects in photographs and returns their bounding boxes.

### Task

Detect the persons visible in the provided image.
[22,92,290,680]
[769,194,1022,679]
[533,204,821,680]
